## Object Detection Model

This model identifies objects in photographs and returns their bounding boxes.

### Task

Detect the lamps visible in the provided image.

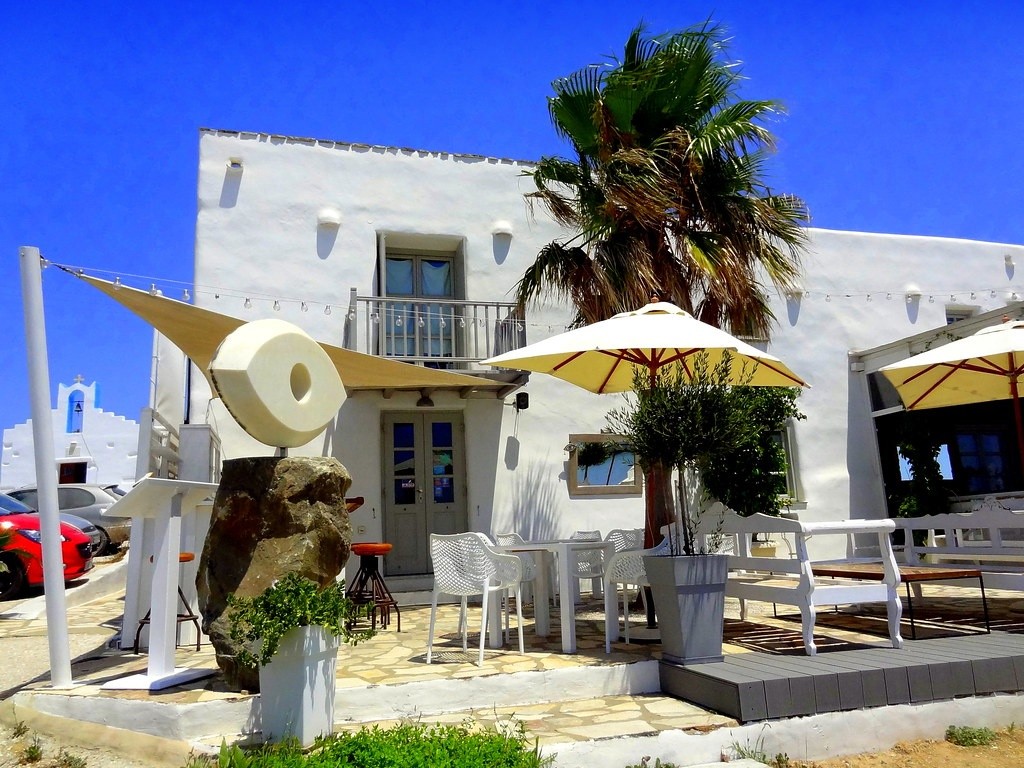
[785,283,802,294]
[496,222,513,236]
[417,392,434,407]
[320,208,342,225]
[904,285,922,299]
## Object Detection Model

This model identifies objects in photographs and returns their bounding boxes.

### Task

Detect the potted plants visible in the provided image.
[604,340,806,665]
[898,405,944,515]
[227,572,377,753]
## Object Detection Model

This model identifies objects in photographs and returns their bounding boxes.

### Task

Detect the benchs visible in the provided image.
[882,495,1024,609]
[687,501,905,656]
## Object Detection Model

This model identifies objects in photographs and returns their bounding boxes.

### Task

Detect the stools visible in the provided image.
[344,541,402,635]
[135,554,201,654]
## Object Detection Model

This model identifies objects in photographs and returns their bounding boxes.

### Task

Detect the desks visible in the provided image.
[769,563,991,640]
[485,539,619,658]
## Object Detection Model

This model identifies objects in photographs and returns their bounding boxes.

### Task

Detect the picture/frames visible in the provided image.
[568,435,644,496]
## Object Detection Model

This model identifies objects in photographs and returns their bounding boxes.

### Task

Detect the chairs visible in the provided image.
[426,528,764,667]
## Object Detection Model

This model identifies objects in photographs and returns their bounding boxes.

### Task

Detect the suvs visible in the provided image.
[3,482,133,557]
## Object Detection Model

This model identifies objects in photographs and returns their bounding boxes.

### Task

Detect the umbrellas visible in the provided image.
[480,296,810,545]
[879,315,1024,460]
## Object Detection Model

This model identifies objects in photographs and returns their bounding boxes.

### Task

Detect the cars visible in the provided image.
[433,463,455,504]
[0,491,102,603]
